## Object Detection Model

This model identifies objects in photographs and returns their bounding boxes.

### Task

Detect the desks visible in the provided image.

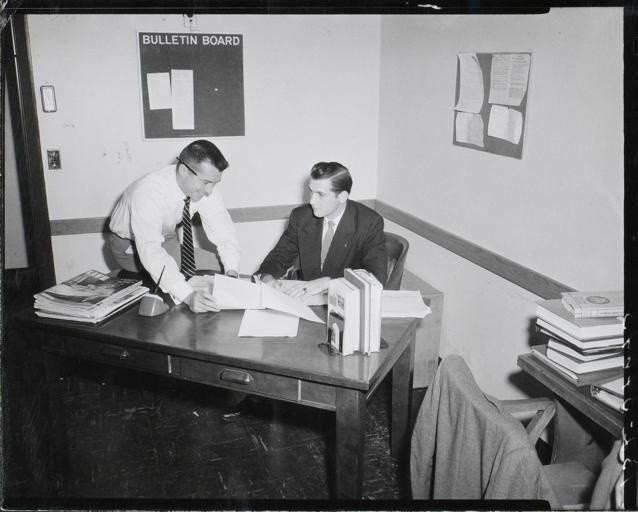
[516,354,625,464]
[9,269,431,500]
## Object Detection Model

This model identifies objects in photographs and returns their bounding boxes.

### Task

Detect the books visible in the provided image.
[325,267,384,356]
[32,269,151,325]
[531,289,625,415]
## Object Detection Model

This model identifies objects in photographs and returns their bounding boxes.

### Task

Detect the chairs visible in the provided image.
[287,231,409,290]
[408,353,611,511]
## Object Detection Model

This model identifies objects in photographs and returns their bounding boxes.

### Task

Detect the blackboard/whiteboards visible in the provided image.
[136,30,247,141]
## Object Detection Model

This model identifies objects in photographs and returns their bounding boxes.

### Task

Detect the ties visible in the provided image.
[320,221,335,272]
[181,197,196,280]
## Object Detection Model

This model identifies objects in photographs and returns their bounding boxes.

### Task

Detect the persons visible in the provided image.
[219,162,389,422]
[107,140,242,315]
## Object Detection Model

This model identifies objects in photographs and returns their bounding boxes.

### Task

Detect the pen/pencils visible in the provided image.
[176,157,198,176]
